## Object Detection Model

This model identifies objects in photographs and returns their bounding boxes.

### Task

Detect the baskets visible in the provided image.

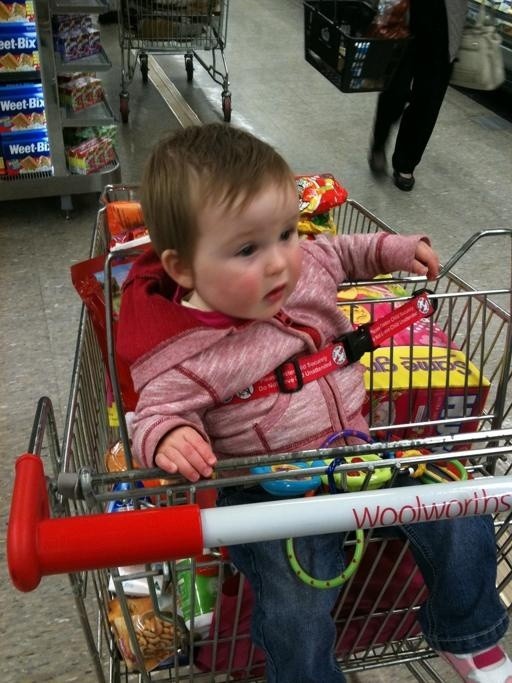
[303,0,412,93]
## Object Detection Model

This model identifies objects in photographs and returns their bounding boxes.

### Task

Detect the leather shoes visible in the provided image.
[393,171,415,191]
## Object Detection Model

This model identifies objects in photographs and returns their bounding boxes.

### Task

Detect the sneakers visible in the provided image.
[435,644,512,683]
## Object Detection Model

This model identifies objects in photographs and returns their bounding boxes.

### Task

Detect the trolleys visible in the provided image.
[111,0,236,128]
[3,177,512,683]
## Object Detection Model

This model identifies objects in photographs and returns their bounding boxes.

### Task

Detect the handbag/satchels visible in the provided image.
[449,0,506,90]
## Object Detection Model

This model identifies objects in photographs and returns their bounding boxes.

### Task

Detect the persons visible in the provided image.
[364,0,470,195]
[115,121,512,683]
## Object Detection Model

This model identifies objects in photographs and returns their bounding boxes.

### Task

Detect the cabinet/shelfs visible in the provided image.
[0,0,122,218]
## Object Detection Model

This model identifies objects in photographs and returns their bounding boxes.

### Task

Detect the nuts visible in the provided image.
[128,602,183,660]
